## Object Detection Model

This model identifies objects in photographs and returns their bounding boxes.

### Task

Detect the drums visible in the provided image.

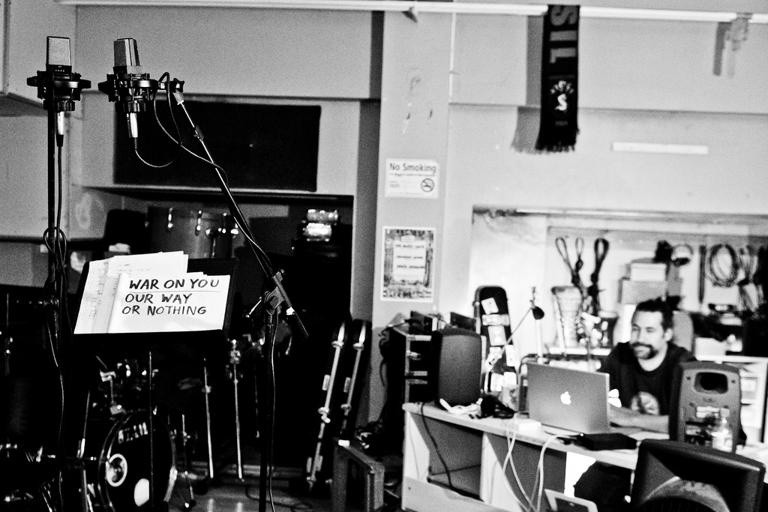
[59,408,178,509]
[144,207,234,260]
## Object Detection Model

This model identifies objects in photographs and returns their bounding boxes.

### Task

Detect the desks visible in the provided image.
[399,400,768,512]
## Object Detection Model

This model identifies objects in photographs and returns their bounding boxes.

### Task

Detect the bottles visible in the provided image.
[710,408,733,453]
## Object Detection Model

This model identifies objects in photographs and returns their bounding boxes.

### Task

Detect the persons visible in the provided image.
[572,299,699,512]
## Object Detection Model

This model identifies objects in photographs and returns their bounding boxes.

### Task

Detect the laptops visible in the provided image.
[525,362,644,443]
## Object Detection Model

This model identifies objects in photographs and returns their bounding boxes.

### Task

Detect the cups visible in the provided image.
[500,385,518,412]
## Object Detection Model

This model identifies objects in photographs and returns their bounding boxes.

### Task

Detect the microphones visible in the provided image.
[113,37,143,150]
[46,36,73,147]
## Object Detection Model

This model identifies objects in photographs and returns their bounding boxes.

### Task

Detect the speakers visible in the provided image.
[669,362,742,456]
[427,327,483,411]
[628,439,766,512]
[113,100,322,193]
